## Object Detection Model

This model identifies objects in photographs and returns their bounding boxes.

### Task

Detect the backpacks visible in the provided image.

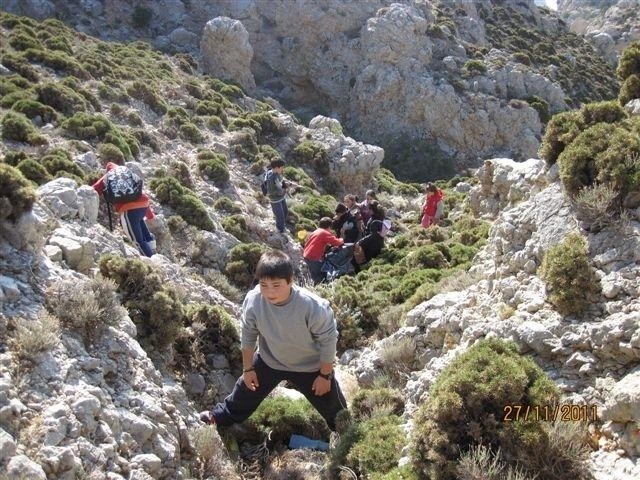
[103,166,143,203]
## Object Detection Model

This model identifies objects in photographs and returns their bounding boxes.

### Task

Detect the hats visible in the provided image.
[336,204,347,213]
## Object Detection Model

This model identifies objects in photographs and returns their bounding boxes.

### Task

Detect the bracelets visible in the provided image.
[319,369,335,380]
[242,367,256,374]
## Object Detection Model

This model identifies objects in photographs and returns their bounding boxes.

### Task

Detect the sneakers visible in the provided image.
[146,240,156,249]
[199,410,217,426]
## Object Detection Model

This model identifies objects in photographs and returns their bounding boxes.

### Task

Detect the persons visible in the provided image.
[92,161,158,256]
[198,250,347,435]
[303,189,390,286]
[420,182,443,229]
[264,158,294,233]
